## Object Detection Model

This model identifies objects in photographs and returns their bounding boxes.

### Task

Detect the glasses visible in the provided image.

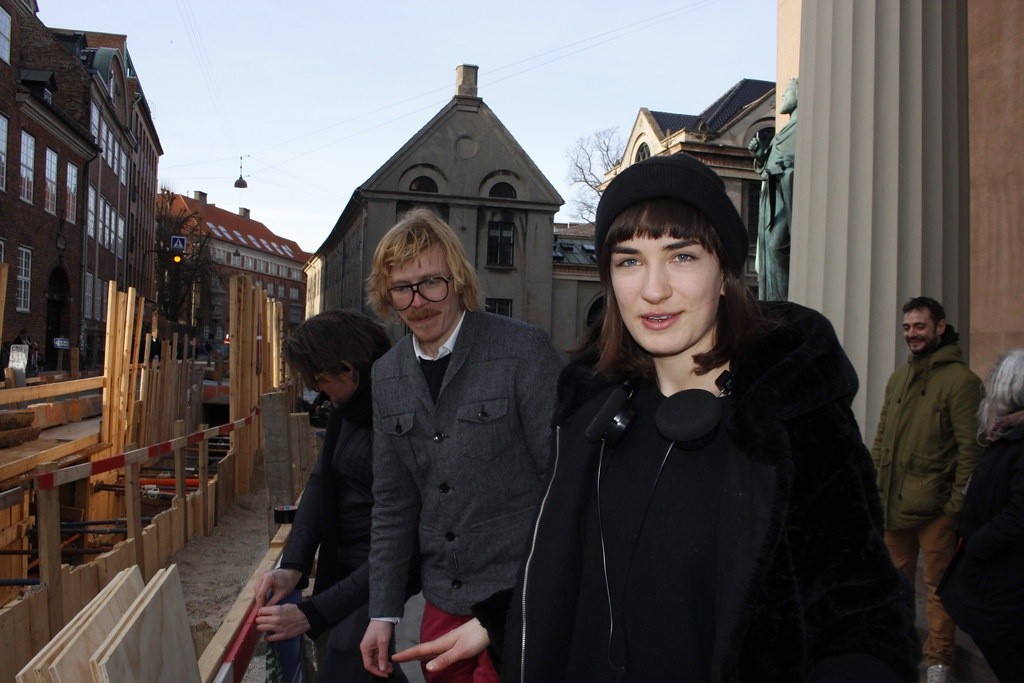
[307,377,322,393]
[385,274,454,312]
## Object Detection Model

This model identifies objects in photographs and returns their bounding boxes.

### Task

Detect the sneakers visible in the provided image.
[926,664,951,683]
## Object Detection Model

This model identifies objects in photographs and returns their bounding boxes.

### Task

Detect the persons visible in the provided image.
[964,347,1024,683]
[132,335,199,362]
[389,149,912,683]
[252,309,393,683]
[11,328,40,375]
[358,204,570,682]
[872,293,988,683]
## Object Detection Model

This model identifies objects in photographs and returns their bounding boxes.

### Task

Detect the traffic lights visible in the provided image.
[159,249,192,271]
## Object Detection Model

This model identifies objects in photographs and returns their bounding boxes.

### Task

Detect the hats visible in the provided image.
[595,153,752,269]
